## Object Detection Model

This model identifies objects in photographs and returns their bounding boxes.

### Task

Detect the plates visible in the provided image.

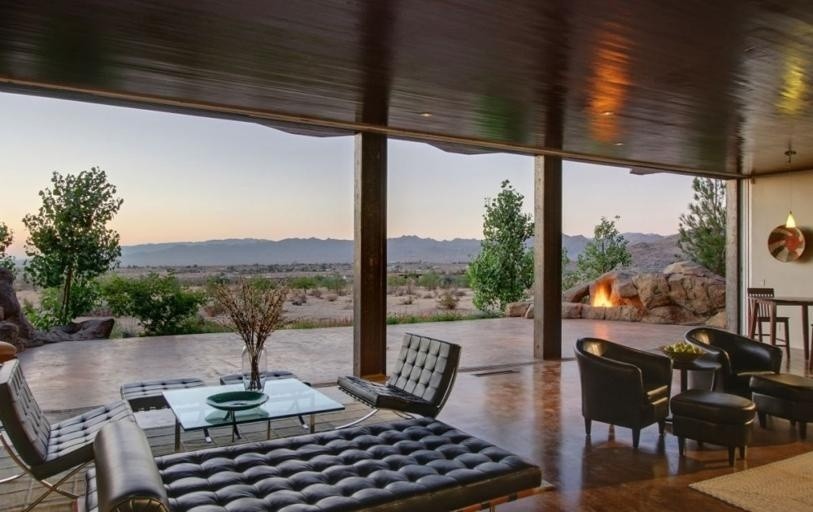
[206,390,269,412]
[767,225,805,263]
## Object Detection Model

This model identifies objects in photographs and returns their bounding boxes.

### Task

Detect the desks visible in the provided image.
[652,347,723,424]
[754,296,811,363]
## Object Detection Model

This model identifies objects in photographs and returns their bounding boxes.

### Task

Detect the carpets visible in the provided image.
[687,448,813,511]
[1,403,499,511]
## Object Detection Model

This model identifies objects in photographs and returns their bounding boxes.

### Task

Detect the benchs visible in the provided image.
[82,418,555,509]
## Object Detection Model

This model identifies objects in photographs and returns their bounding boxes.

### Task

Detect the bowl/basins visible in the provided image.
[662,345,708,363]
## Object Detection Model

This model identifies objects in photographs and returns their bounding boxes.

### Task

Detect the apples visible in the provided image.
[666,341,699,353]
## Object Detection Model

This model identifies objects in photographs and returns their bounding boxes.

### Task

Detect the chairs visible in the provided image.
[751,289,790,351]
[574,337,672,448]
[1,360,135,512]
[336,336,465,428]
[685,326,782,430]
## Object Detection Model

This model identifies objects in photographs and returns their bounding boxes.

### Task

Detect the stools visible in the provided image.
[667,388,755,468]
[121,369,302,404]
[749,372,813,429]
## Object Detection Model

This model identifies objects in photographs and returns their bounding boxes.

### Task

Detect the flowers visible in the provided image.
[209,277,295,346]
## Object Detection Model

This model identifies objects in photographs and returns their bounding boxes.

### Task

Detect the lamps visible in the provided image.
[784,150,798,230]
